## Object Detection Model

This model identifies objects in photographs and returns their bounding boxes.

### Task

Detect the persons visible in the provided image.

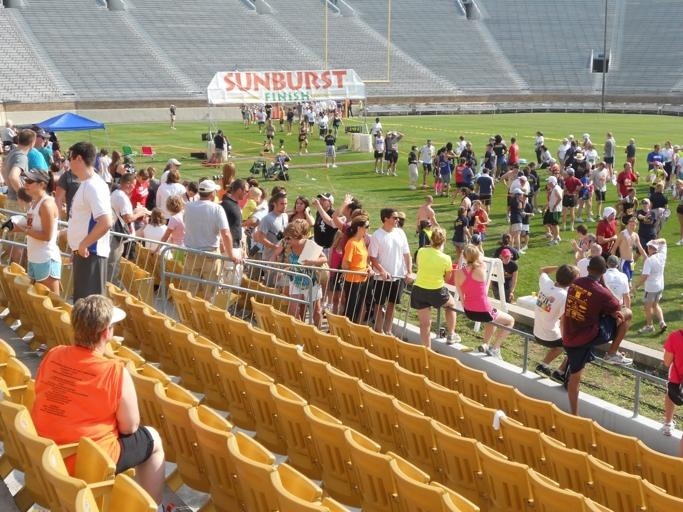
[409,132,682,437]
[239,99,363,145]
[169,105,177,130]
[31,294,178,512]
[0,121,413,336]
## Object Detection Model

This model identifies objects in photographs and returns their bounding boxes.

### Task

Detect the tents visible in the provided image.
[38,112,108,148]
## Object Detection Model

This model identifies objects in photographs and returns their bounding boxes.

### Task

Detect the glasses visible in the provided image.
[390,216,400,220]
[362,224,370,229]
[23,178,41,184]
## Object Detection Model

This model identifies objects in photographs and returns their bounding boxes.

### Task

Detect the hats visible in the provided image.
[512,187,525,195]
[518,175,528,182]
[95,304,128,333]
[197,179,221,193]
[36,130,52,139]
[167,158,182,166]
[317,192,335,205]
[21,168,51,185]
[545,175,558,185]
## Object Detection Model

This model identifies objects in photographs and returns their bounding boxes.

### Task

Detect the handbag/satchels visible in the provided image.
[315,261,331,284]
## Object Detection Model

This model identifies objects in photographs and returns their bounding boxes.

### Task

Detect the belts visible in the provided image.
[73,250,93,257]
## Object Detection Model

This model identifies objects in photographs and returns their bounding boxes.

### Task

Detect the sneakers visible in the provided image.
[447,334,462,345]
[560,211,603,232]
[637,325,656,334]
[477,343,503,360]
[534,364,551,379]
[658,320,667,334]
[549,369,565,385]
[662,421,675,437]
[533,208,543,214]
[517,244,528,255]
[543,231,561,247]
[675,238,683,246]
[602,350,634,366]
